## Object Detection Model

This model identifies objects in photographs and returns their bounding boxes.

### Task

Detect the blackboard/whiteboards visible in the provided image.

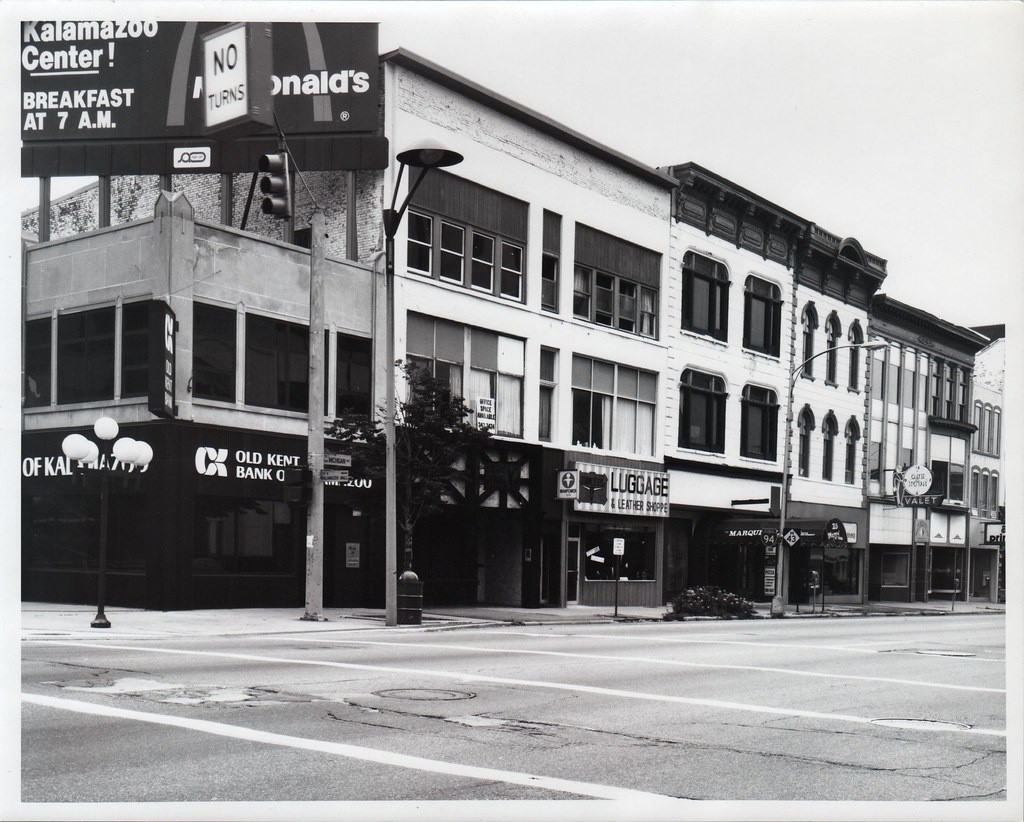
[612,537,625,556]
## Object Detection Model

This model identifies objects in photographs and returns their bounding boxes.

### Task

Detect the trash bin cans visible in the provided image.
[397,580,423,624]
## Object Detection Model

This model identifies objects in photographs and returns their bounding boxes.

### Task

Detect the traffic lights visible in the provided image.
[258,151,294,220]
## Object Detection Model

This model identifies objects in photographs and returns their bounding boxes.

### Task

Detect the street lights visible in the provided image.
[376,144,466,628]
[767,340,887,621]
[60,415,154,628]
